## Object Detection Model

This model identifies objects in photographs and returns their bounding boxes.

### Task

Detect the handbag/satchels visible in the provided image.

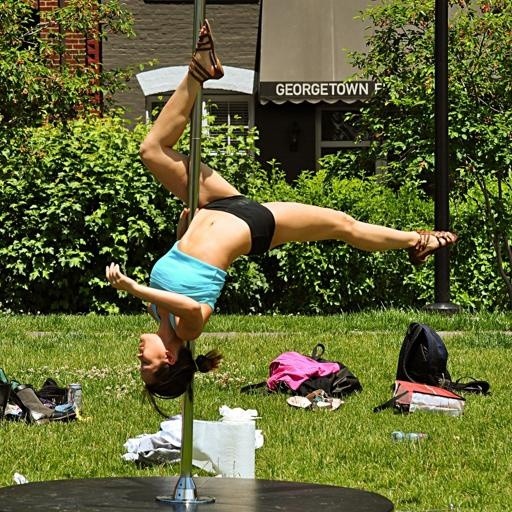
[266,354,363,398]
[373,380,467,417]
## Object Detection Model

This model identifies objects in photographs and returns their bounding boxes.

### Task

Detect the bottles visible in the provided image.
[66,383,82,408]
[391,431,429,442]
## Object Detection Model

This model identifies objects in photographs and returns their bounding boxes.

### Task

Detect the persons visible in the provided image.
[105,18,459,420]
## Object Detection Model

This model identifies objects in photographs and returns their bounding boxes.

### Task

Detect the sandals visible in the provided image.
[187,18,224,90]
[404,230,458,264]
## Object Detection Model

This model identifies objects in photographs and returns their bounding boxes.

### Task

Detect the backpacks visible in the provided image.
[396,322,453,388]
